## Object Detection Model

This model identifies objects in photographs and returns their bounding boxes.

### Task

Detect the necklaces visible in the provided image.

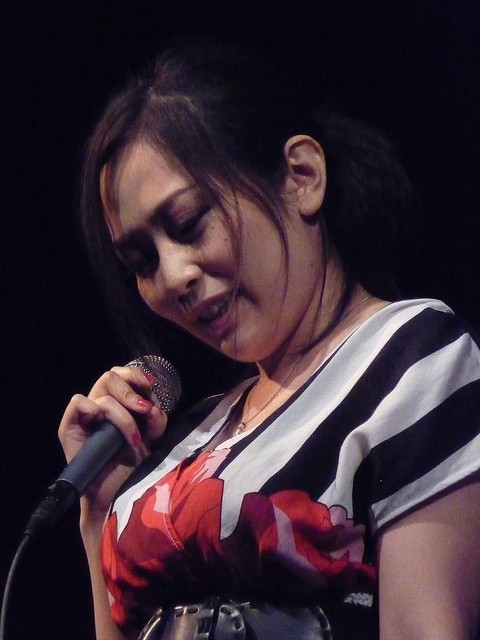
[232,293,372,434]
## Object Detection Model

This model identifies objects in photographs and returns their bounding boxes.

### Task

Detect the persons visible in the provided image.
[56,35,480,639]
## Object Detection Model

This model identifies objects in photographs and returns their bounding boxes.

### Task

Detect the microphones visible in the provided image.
[25,355,180,538]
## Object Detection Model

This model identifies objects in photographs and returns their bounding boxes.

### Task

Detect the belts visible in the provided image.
[135,597,332,640]
